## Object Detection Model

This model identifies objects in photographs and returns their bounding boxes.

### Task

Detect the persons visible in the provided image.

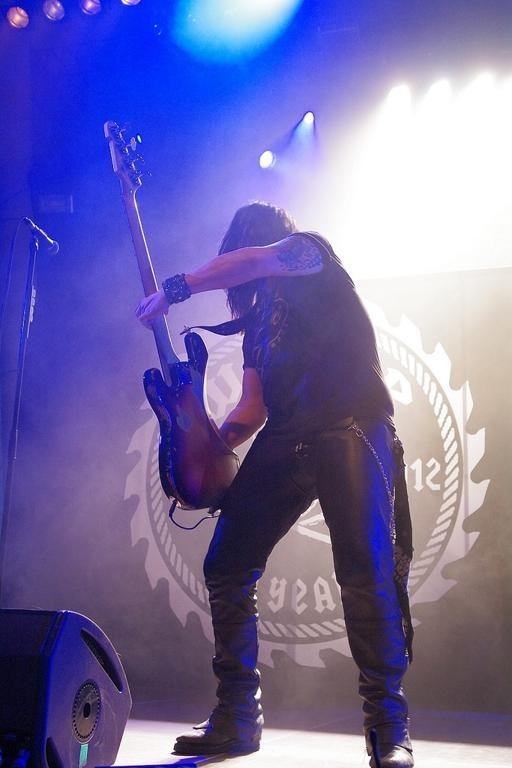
[133,200,420,767]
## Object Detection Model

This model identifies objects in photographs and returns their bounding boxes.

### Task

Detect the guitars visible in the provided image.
[103,118,240,511]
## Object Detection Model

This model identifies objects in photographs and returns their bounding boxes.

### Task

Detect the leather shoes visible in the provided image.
[362,722,415,768]
[171,716,261,755]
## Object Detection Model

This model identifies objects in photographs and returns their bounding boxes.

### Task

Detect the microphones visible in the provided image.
[25,216,63,259]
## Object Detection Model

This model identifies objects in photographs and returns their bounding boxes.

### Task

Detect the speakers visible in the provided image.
[1,608,134,767]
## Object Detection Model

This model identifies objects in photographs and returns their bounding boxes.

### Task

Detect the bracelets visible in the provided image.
[162,271,191,304]
